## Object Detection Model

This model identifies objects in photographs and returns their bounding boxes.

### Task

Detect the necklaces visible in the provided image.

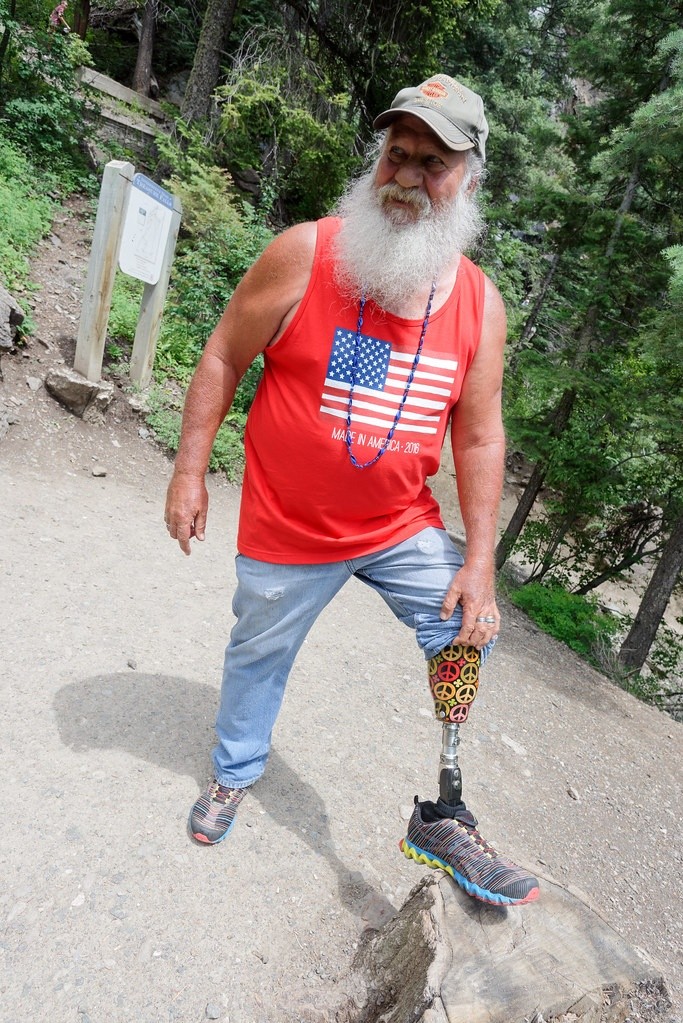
[346,265,435,468]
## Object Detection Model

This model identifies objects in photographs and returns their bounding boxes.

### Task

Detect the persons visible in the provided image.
[162,70,539,907]
[49,0,71,30]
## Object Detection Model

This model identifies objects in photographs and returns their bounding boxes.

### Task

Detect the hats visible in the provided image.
[373,74,488,163]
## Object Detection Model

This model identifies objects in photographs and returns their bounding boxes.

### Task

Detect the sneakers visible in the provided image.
[190,777,254,844]
[400,796,540,906]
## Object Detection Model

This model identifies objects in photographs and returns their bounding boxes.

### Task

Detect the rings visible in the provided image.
[475,617,486,624]
[486,618,494,622]
